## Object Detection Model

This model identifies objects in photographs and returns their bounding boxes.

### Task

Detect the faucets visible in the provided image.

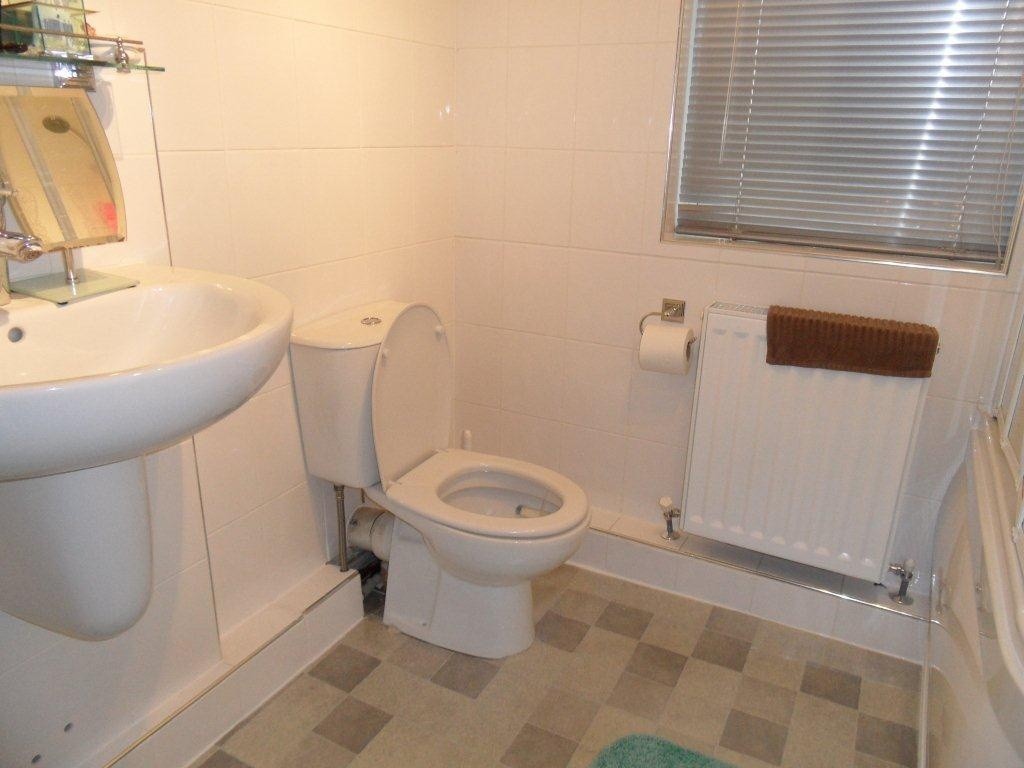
[1,173,46,308]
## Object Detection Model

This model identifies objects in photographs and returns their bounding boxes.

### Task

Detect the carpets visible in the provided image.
[588,733,739,768]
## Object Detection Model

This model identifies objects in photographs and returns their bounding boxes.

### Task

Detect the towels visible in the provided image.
[763,298,943,381]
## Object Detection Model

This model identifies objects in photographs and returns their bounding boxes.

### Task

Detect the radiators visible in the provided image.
[677,299,937,585]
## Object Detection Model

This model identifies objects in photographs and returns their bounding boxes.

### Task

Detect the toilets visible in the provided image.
[287,299,595,660]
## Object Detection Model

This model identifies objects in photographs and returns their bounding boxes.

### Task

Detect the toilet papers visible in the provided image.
[635,321,695,380]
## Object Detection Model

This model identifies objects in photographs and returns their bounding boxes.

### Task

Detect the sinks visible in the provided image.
[1,264,298,493]
